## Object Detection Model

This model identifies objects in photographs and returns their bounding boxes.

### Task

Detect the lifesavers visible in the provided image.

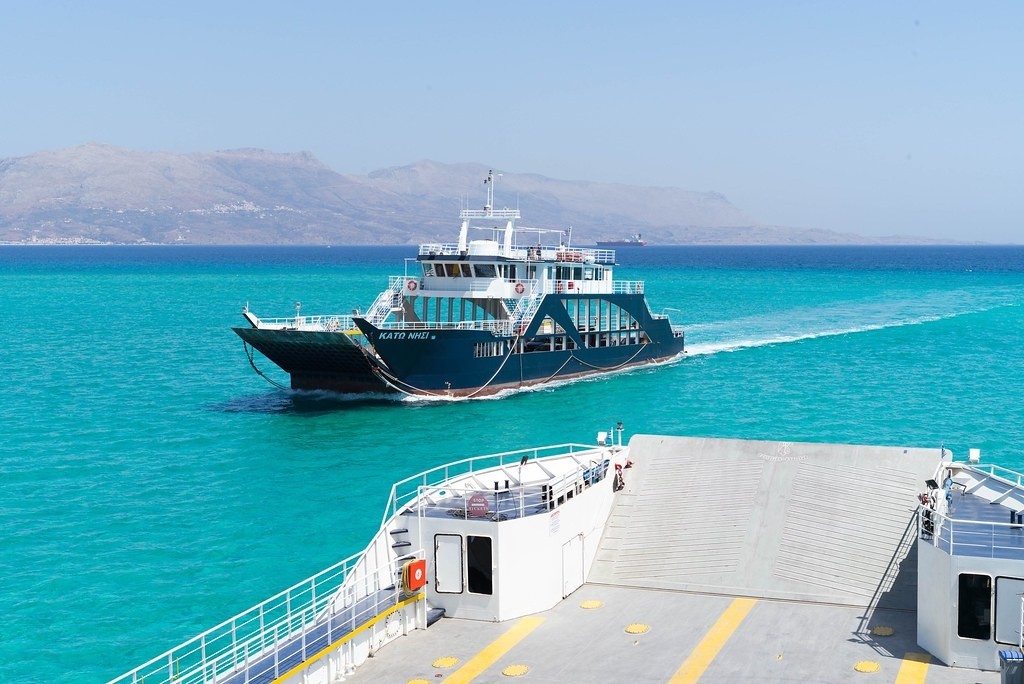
[515,283,525,294]
[407,280,418,291]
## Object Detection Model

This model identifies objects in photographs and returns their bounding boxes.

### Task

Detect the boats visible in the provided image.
[230,169,688,397]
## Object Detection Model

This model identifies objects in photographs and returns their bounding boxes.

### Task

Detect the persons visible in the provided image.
[559,241,565,252]
[527,246,536,261]
[536,243,543,260]
